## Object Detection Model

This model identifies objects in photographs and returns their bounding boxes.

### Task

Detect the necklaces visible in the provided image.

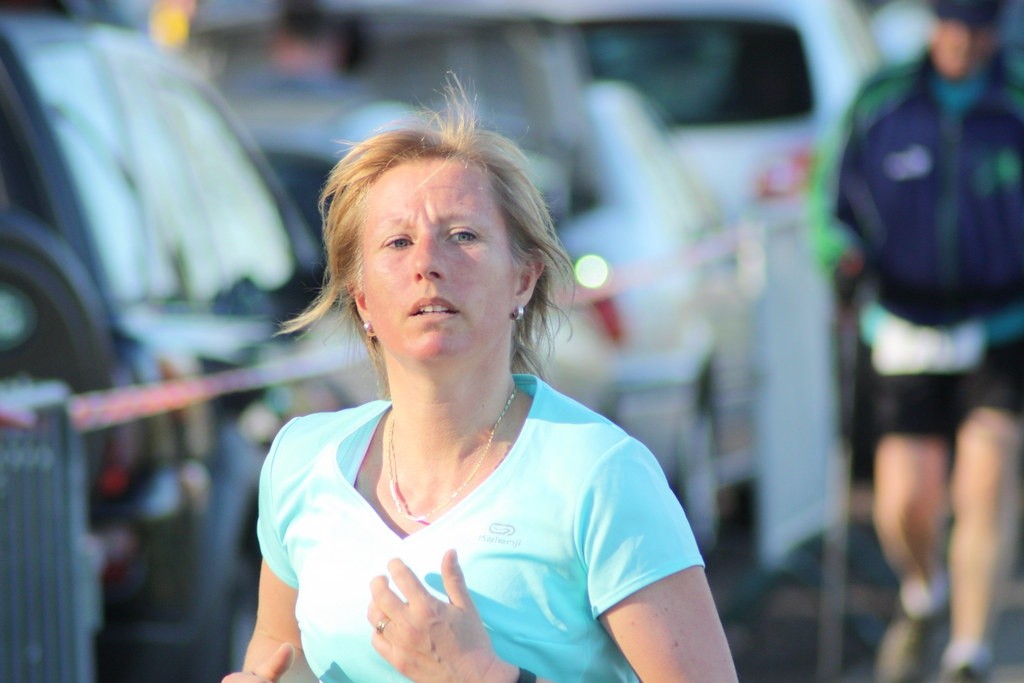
[387,385,518,522]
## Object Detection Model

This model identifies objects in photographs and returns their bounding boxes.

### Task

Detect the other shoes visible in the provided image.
[873,589,950,683]
[937,654,994,683]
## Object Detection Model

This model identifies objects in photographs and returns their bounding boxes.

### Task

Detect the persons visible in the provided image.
[806,0,1024,682]
[220,125,743,683]
[243,0,360,104]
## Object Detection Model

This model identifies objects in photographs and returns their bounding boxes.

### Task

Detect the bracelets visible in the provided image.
[517,667,536,683]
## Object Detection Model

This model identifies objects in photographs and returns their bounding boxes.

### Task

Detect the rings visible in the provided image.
[377,618,388,632]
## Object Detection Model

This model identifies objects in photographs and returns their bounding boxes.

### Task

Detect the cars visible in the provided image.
[0,2,934,622]
